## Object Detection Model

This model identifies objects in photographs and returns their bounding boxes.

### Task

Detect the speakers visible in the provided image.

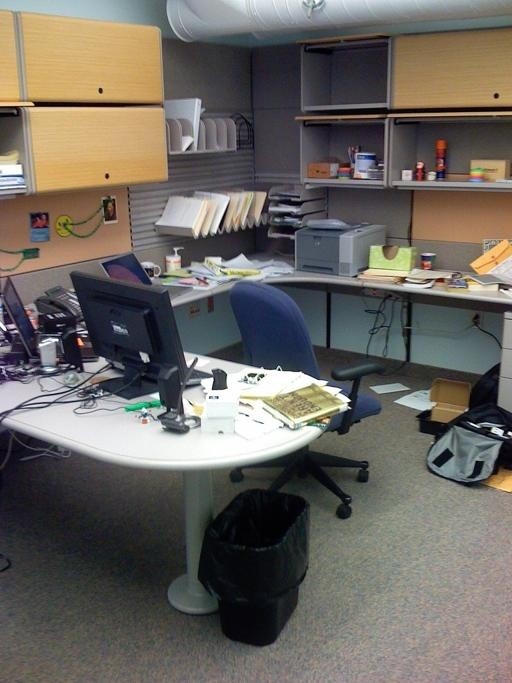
[158,364,184,414]
[61,329,84,373]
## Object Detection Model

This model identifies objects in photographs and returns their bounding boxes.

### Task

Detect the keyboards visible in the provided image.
[185,368,212,387]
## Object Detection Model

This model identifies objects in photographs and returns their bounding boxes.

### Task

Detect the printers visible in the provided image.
[294,218,387,277]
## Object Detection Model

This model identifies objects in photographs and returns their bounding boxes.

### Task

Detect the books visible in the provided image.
[155,191,267,238]
[356,270,511,291]
[262,383,348,430]
[0,151,27,195]
[165,98,201,152]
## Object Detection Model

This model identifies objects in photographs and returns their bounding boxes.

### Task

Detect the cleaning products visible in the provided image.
[172,247,184,270]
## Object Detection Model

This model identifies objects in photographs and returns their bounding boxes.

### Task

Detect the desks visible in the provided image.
[152,251,512,308]
[0,324,332,615]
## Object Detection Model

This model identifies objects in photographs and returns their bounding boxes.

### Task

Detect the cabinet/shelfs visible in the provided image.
[22,106,168,193]
[294,33,393,113]
[293,114,389,187]
[1,9,23,103]
[0,102,32,199]
[388,112,512,192]
[391,27,512,111]
[16,10,165,105]
[497,311,512,418]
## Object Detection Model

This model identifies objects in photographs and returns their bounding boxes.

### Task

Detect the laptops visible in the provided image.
[98,250,193,301]
[1,275,99,360]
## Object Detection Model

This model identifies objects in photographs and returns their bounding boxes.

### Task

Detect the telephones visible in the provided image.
[34,284,85,322]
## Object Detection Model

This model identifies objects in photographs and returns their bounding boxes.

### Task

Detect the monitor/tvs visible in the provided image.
[68,270,189,400]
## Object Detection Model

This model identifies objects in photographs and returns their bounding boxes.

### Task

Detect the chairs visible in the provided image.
[229,281,388,520]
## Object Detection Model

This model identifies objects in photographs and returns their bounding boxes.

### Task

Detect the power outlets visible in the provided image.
[467,310,484,329]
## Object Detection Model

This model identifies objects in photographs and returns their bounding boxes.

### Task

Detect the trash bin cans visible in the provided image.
[196,488,311,648]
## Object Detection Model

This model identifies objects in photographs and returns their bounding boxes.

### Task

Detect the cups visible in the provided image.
[420,253,435,270]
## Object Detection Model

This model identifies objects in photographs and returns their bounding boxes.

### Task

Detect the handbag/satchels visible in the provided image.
[425,400,512,484]
